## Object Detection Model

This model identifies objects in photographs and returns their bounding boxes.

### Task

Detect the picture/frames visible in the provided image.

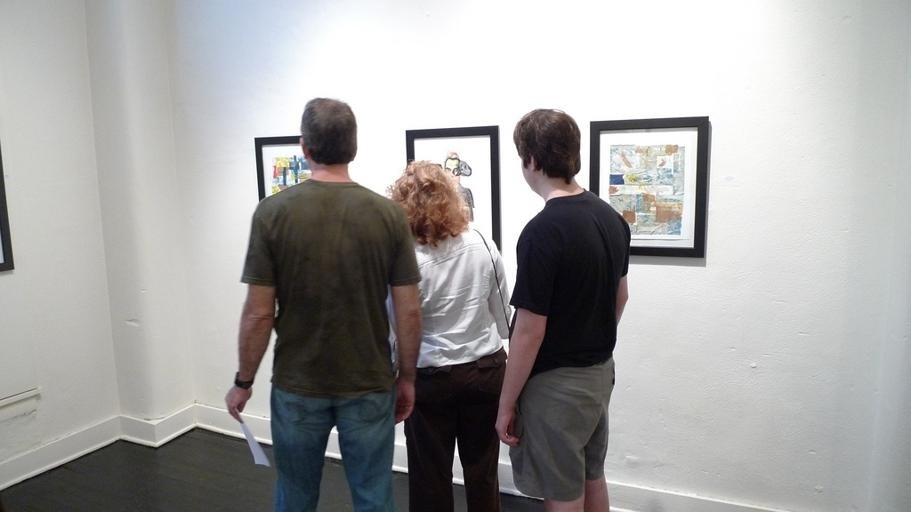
[252,134,312,206]
[402,127,500,263]
[587,115,709,262]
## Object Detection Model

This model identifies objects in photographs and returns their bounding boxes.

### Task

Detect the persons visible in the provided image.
[391,160,512,512]
[494,107,630,512]
[224,95,423,512]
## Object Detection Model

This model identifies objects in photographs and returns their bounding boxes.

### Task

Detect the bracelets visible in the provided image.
[232,373,254,389]
